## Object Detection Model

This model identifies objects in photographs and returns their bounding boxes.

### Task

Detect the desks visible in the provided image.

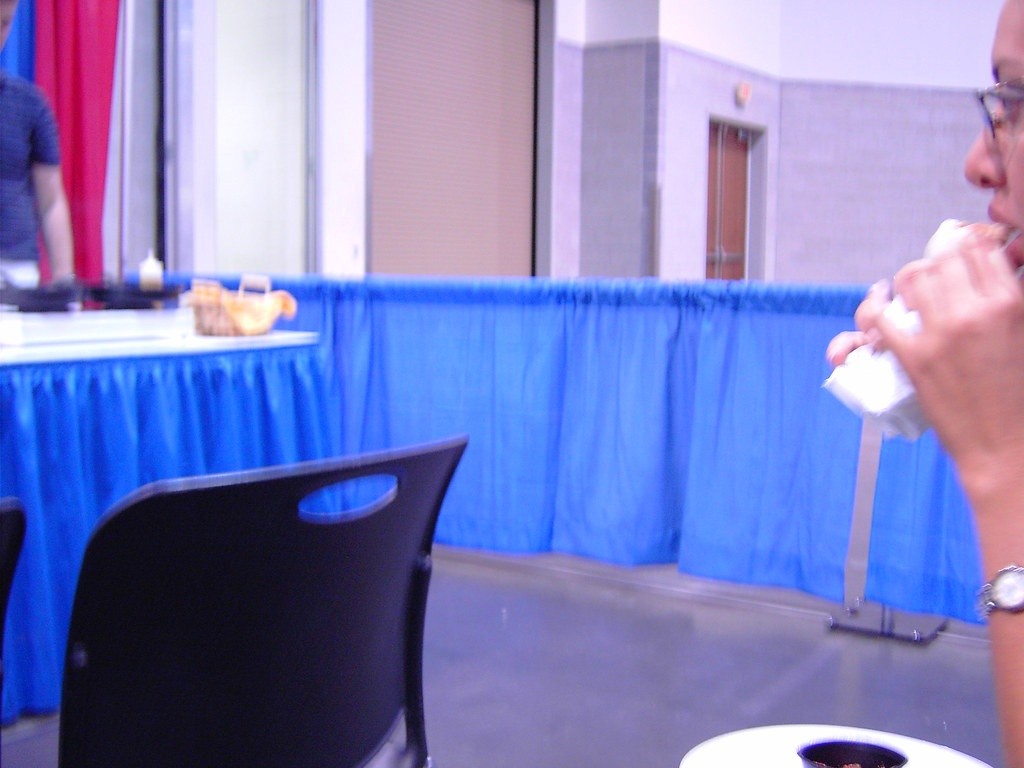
[0,303,348,728]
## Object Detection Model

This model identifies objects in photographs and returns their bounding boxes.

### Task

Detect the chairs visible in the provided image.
[57,431,471,768]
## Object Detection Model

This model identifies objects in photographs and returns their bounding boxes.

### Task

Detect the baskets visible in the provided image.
[193,277,280,333]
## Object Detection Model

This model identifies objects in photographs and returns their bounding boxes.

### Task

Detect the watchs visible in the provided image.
[977,565,1024,618]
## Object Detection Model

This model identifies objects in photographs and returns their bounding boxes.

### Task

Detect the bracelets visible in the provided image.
[66,273,75,280]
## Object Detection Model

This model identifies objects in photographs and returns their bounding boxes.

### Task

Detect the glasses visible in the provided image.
[972,76,1024,138]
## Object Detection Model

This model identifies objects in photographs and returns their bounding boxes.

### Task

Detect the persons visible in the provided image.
[825,0,1024,768]
[0,0,80,310]
